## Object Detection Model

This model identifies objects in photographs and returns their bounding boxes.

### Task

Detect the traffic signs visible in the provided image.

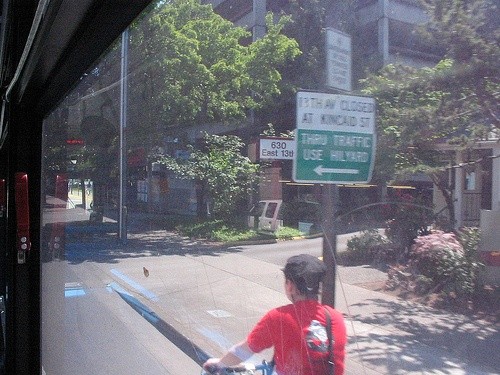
[290,86,377,186]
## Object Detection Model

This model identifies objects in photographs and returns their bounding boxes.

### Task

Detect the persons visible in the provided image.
[202,252,348,375]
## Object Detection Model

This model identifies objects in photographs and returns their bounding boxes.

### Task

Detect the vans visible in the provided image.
[248,199,286,233]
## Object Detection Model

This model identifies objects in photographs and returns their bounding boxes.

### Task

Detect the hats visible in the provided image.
[280,254,328,289]
